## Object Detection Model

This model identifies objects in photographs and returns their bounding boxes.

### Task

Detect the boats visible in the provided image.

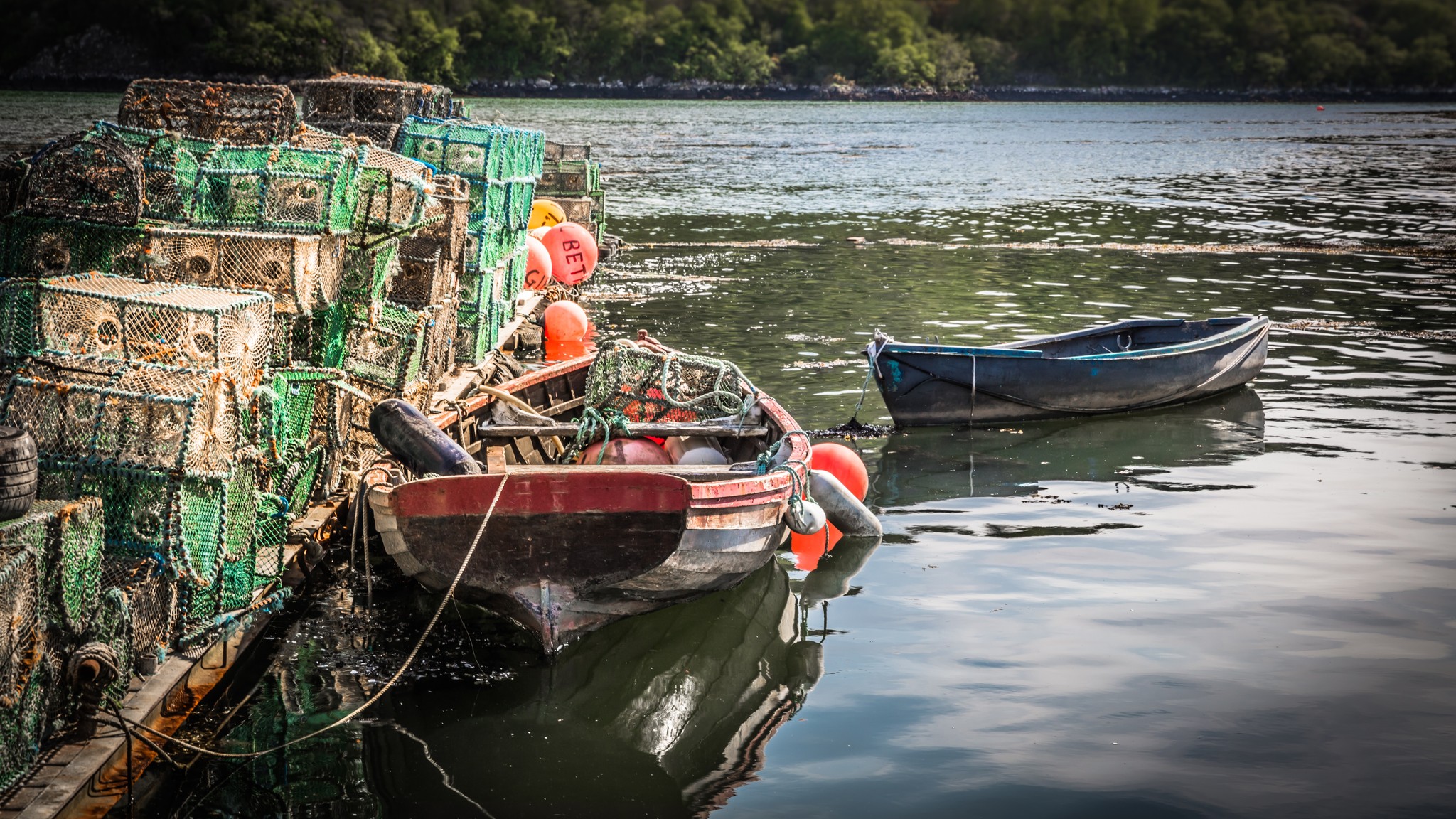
[861,307,1260,435]
[356,326,816,670]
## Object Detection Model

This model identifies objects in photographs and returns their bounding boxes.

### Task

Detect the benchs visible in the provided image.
[477,421,767,437]
